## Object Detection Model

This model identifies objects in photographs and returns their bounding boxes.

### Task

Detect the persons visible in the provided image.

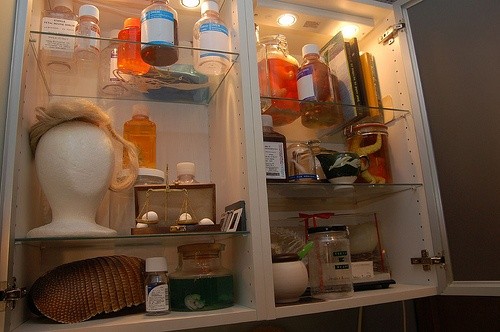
[26,99,139,238]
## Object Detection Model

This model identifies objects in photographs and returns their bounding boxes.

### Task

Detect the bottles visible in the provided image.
[109,170,164,232]
[144,256,171,316]
[192,1,232,76]
[343,123,394,185]
[38,0,76,75]
[168,242,235,312]
[97,29,130,97]
[140,0,179,67]
[306,224,354,300]
[117,17,150,76]
[74,4,102,62]
[122,104,157,169]
[174,162,199,183]
[253,23,273,112]
[272,260,309,304]
[259,33,301,127]
[262,114,289,184]
[295,42,340,130]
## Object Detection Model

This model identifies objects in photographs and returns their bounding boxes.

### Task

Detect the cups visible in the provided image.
[317,151,370,189]
[287,143,317,183]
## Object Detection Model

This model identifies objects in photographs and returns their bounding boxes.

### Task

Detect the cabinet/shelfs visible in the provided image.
[0,0,500,332]
